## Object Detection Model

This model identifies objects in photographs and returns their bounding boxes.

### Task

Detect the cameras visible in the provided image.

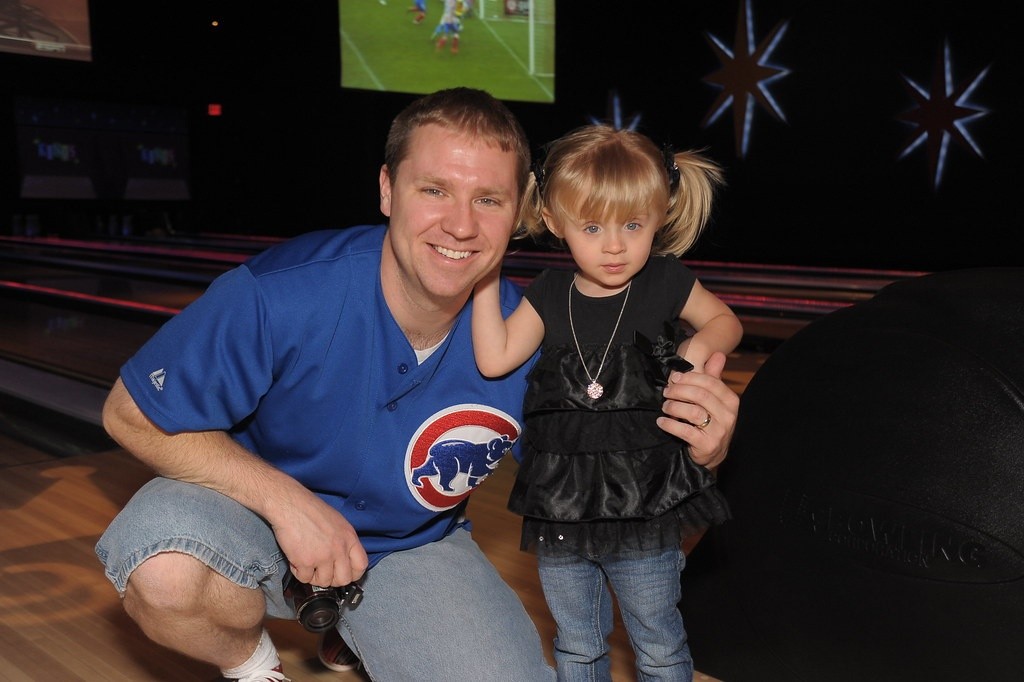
[281,568,364,634]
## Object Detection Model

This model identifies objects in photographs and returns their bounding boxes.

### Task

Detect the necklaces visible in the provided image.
[568,273,632,399]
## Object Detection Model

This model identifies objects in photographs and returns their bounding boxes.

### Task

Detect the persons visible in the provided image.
[94,87,740,682]
[431,0,468,55]
[407,0,426,24]
[471,125,744,682]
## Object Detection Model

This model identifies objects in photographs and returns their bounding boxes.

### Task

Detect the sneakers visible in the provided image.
[226,661,291,682]
[318,623,362,672]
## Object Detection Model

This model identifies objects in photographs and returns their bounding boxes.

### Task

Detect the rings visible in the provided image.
[697,413,711,428]
